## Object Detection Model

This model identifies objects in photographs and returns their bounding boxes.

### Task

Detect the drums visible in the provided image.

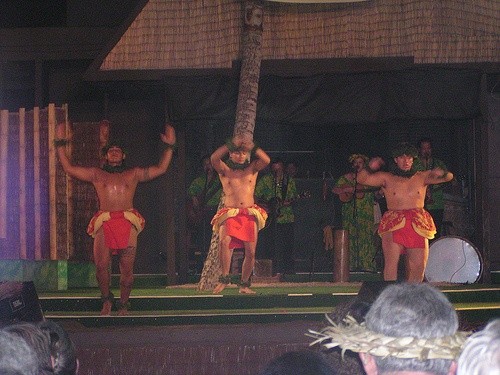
[424,235,484,284]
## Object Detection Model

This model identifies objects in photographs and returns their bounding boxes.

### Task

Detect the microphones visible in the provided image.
[355,166,359,169]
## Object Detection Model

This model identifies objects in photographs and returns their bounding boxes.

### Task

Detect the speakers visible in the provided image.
[0,280,45,329]
[342,280,404,326]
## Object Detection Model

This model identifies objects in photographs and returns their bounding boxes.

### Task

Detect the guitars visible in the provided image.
[338,183,382,201]
[253,190,311,228]
[187,200,220,225]
[424,169,472,205]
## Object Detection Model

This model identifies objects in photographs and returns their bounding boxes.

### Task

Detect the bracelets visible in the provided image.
[253,145,259,153]
[365,160,374,173]
[163,143,177,150]
[55,139,64,146]
[225,138,237,151]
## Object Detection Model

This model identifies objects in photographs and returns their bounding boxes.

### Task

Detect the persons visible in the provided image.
[0,320,79,375]
[186,155,222,270]
[414,137,453,245]
[357,142,454,283]
[255,158,300,274]
[263,281,500,375]
[210,135,271,293]
[335,153,377,271]
[55,123,176,315]
